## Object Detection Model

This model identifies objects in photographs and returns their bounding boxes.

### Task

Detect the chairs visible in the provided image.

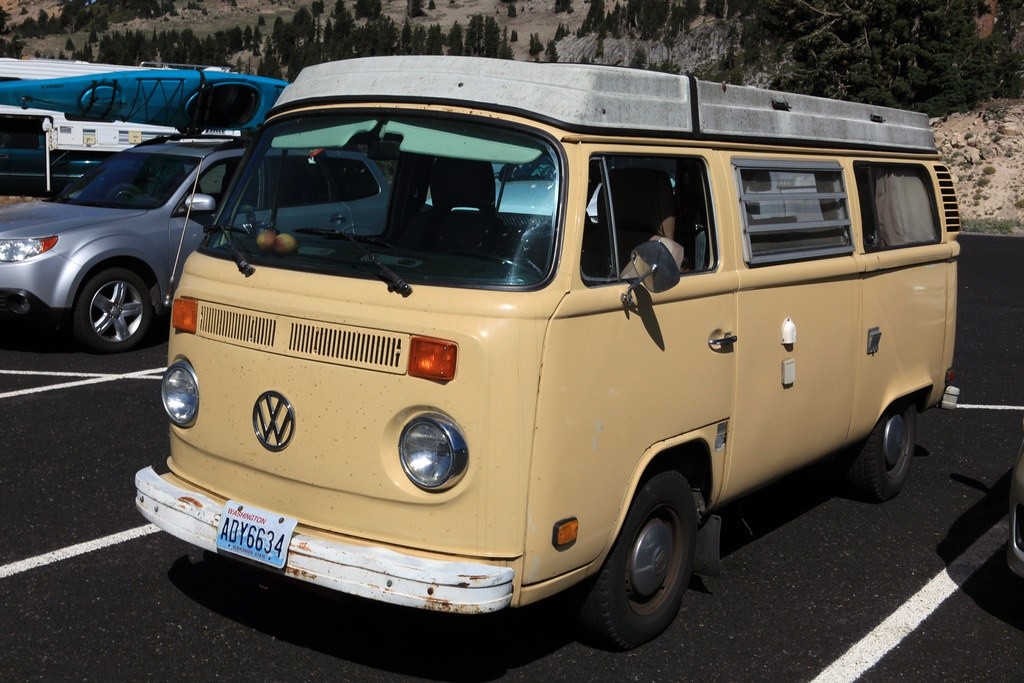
[409,160,502,263]
[597,166,677,272]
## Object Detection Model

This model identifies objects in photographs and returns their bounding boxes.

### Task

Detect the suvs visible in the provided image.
[0,136,390,357]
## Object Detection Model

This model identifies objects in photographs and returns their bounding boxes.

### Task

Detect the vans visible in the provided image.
[133,57,961,656]
